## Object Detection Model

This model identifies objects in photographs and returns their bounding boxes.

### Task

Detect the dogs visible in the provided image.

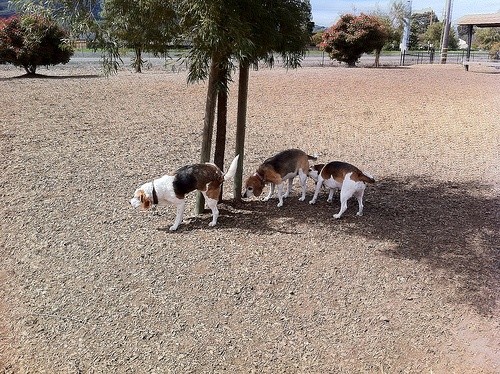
[242,149,318,208]
[307,161,375,219]
[129,153,240,232]
